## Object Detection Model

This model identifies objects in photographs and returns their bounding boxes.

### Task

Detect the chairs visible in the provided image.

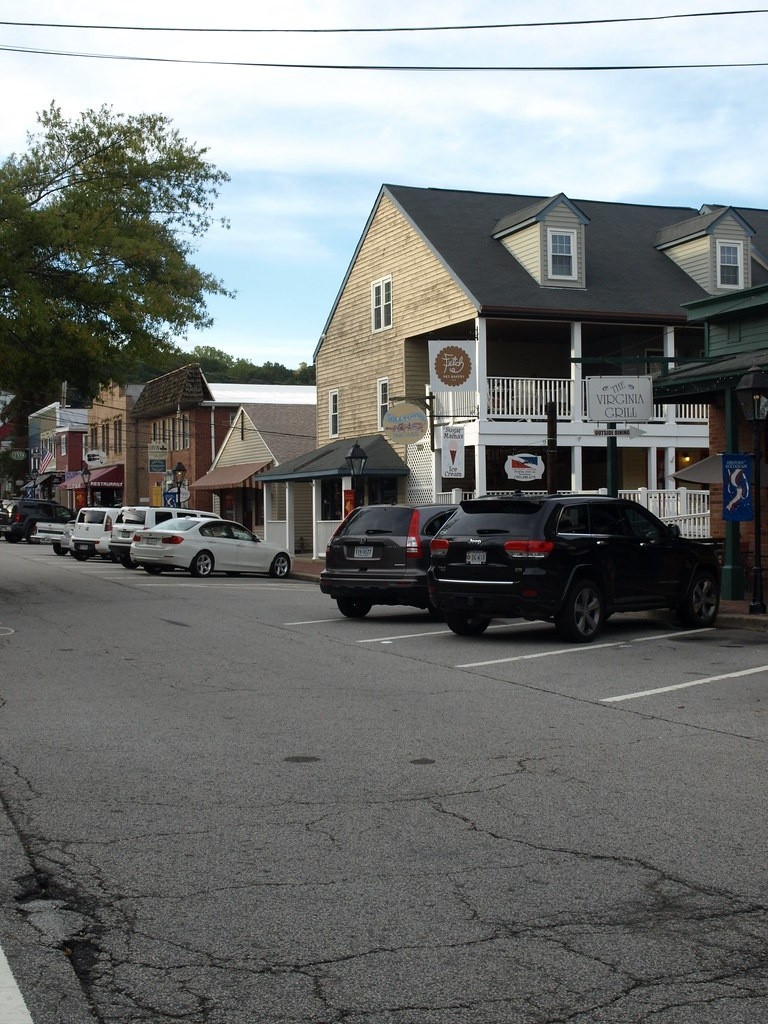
[511,380,539,421]
[486,382,505,415]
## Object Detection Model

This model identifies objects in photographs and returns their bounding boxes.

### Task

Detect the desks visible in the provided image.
[490,389,519,414]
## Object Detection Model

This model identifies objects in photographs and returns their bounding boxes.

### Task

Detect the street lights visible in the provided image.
[31,466,39,498]
[172,462,187,507]
[736,360,768,615]
[345,438,368,509]
[81,467,91,508]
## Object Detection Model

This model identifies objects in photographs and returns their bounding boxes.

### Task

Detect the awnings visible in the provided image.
[253,436,409,482]
[60,465,124,490]
[188,460,273,490]
[24,474,50,488]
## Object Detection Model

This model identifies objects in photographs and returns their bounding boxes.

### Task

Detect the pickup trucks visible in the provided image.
[30,520,75,556]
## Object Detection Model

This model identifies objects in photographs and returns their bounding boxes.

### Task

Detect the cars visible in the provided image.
[130,516,294,578]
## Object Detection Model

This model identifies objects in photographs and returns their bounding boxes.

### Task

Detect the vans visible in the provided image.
[109,505,224,569]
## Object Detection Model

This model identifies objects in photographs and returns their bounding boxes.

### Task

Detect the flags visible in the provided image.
[39,449,53,474]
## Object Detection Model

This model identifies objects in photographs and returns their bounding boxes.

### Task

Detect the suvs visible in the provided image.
[427,494,722,643]
[0,498,76,544]
[68,507,121,559]
[319,501,459,622]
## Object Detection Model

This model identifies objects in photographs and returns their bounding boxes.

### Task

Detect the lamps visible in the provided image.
[683,452,690,462]
